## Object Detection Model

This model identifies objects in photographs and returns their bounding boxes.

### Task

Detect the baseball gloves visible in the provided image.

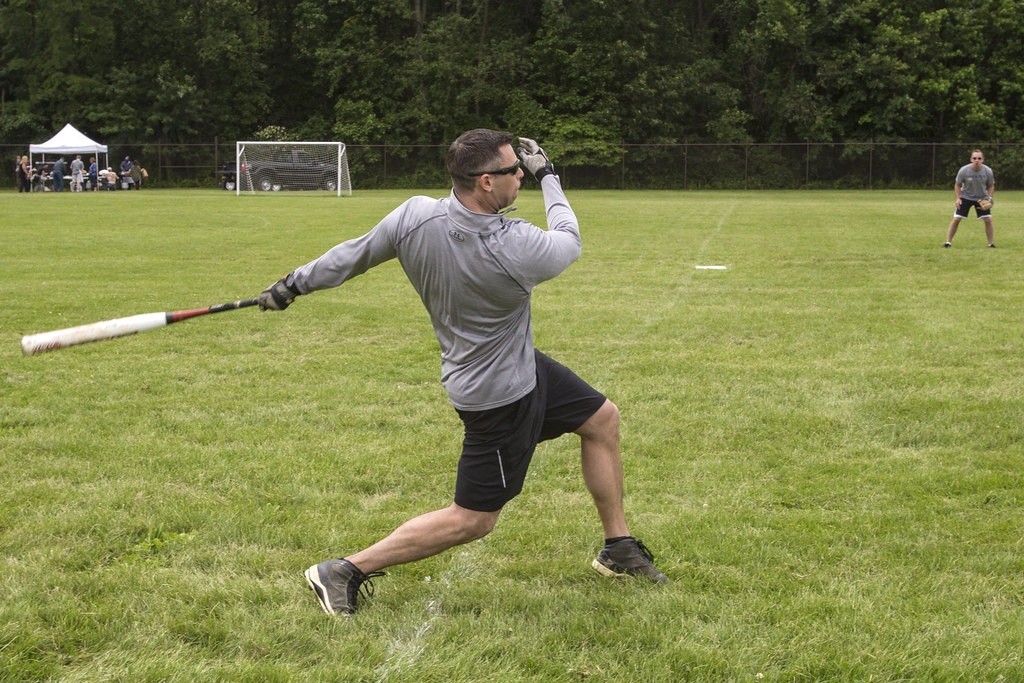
[976,194,993,211]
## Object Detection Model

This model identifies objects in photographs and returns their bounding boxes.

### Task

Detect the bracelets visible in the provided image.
[535,162,556,181]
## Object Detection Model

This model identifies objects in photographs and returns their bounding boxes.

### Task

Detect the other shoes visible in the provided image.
[944,243,951,247]
[989,244,997,247]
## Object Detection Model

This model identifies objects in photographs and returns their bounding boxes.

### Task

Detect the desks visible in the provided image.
[45,176,71,192]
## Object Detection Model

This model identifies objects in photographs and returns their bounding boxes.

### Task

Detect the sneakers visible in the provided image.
[304,558,386,619]
[592,538,670,582]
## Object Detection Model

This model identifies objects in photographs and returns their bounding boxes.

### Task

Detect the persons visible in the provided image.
[257,130,666,619]
[53,157,66,192]
[16,151,51,193]
[105,167,119,191]
[119,156,144,190]
[70,155,84,192]
[88,157,97,191]
[943,149,996,249]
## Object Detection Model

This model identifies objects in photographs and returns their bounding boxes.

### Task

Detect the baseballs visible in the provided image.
[21,295,261,356]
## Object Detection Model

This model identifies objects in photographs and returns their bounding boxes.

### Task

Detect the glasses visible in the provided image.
[972,157,983,160]
[469,160,521,175]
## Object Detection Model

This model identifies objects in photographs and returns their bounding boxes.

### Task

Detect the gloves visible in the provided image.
[259,280,295,311]
[516,137,554,182]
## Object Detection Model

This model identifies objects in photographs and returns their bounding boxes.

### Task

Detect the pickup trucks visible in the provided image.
[220,148,338,191]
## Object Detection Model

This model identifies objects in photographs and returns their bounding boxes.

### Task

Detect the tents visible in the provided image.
[30,124,109,192]
